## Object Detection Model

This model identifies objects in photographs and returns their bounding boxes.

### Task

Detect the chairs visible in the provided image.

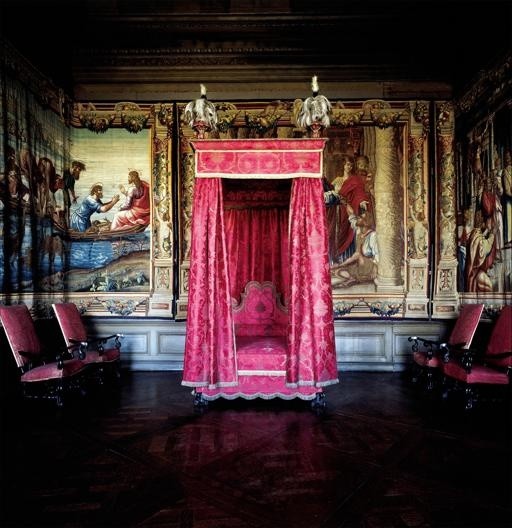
[0,299,124,422]
[407,302,512,417]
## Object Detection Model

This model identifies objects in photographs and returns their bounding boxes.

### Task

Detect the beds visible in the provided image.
[192,282,329,416]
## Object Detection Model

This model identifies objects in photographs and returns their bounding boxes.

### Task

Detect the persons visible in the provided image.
[338,154,375,238]
[331,156,357,264]
[69,183,120,234]
[330,194,378,287]
[457,206,475,248]
[108,168,151,233]
[407,205,429,262]
[1,143,90,296]
[155,206,173,259]
[502,153,511,200]
[463,210,503,292]
